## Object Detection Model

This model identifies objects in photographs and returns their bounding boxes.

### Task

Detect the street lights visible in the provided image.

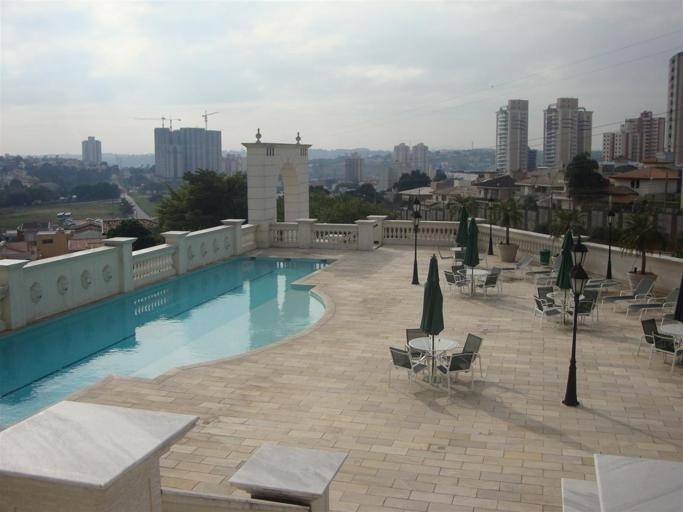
[407,192,422,286]
[605,207,619,281]
[485,195,498,255]
[560,235,588,408]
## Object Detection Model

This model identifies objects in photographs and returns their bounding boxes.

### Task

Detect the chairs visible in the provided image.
[491,254,683,376]
[387,326,485,399]
[435,241,505,302]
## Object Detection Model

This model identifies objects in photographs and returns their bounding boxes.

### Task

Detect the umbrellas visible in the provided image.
[455,207,468,251]
[464,217,479,296]
[420,254,445,377]
[556,229,574,324]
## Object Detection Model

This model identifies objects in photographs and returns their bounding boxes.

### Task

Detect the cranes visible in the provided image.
[131,115,183,131]
[201,108,220,131]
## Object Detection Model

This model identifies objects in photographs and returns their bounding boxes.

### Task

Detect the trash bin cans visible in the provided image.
[539,248,550,265]
